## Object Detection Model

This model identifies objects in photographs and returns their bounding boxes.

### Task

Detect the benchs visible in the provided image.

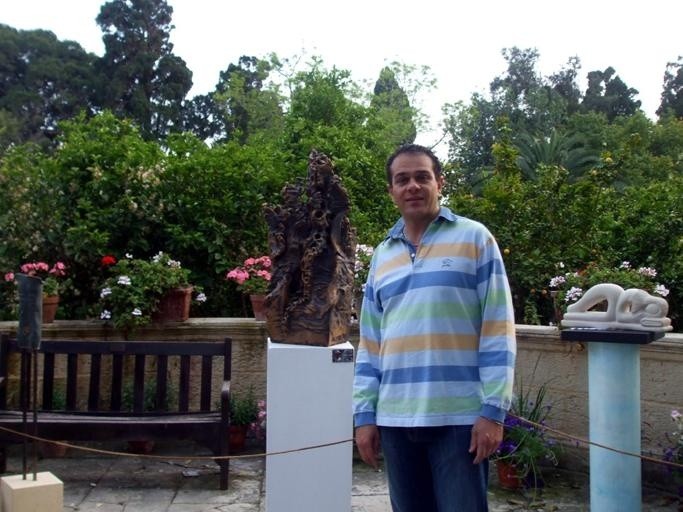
[0,336,230,490]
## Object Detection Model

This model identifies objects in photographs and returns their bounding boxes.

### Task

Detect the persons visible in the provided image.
[351,144,517,512]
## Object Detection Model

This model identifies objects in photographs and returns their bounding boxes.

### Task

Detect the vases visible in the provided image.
[352,289,362,321]
[496,452,533,489]
[550,288,578,330]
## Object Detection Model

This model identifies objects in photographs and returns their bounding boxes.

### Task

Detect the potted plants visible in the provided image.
[30,373,175,457]
[215,386,256,451]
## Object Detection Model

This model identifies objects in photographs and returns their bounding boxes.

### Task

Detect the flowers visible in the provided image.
[543,248,671,332]
[352,245,374,297]
[248,399,267,439]
[4,244,271,332]
[658,407,683,498]
[482,350,560,509]
